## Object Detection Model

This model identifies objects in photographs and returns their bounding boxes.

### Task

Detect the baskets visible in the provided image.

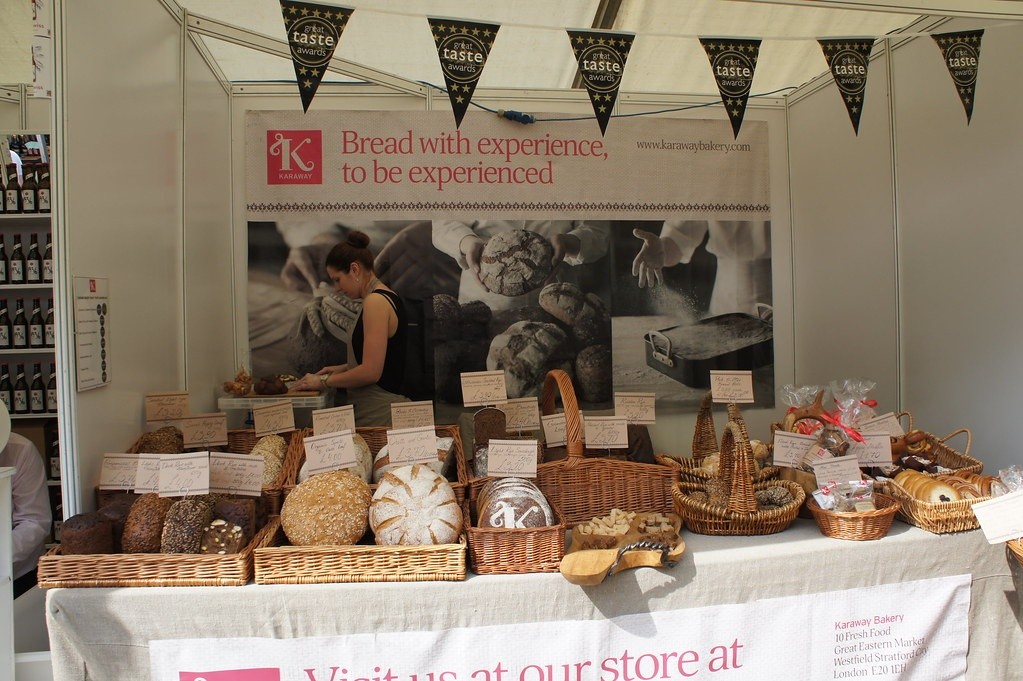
[252,504,468,581]
[882,477,992,535]
[37,514,279,587]
[771,410,984,519]
[806,491,901,541]
[1006,537,1023,622]
[282,425,468,507]
[94,429,301,525]
[670,420,806,536]
[654,387,781,484]
[463,493,568,576]
[469,368,681,530]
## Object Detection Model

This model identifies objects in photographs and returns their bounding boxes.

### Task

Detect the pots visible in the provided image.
[643,301,774,388]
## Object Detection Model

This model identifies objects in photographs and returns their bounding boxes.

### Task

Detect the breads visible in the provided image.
[431,229,613,408]
[60,407,553,557]
[802,430,1007,513]
[684,439,795,515]
[579,507,678,550]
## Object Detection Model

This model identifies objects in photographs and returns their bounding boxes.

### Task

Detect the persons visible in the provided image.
[275,221,444,296]
[0,399,52,600]
[432,221,610,310]
[290,231,425,428]
[632,219,772,321]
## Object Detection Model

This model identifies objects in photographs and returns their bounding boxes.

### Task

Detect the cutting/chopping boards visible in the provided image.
[242,390,319,398]
[560,512,685,586]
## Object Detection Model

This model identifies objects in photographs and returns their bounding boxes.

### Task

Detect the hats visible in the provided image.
[0,399,12,453]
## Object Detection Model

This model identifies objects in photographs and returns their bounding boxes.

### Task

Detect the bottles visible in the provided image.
[48,361,58,413]
[13,297,29,348]
[0,233,53,284]
[0,298,12,349]
[30,298,43,348]
[10,134,40,156]
[49,431,61,480]
[0,161,50,214]
[14,363,29,413]
[31,362,46,412]
[0,362,13,414]
[53,492,63,543]
[44,299,55,348]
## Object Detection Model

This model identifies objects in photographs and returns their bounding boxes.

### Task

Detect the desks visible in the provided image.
[44,517,1023,681]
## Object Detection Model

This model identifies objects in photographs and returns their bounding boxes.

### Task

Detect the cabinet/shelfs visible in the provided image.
[0,85,60,548]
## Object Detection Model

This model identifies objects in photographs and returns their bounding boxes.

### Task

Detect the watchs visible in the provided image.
[320,374,330,389]
